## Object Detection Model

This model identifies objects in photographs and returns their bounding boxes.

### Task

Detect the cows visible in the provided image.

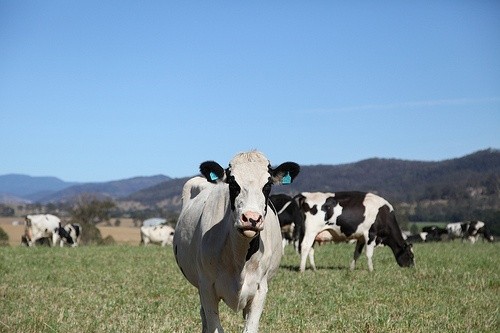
[272,189,492,272]
[138,225,175,248]
[173,149,301,333]
[21,213,82,248]
